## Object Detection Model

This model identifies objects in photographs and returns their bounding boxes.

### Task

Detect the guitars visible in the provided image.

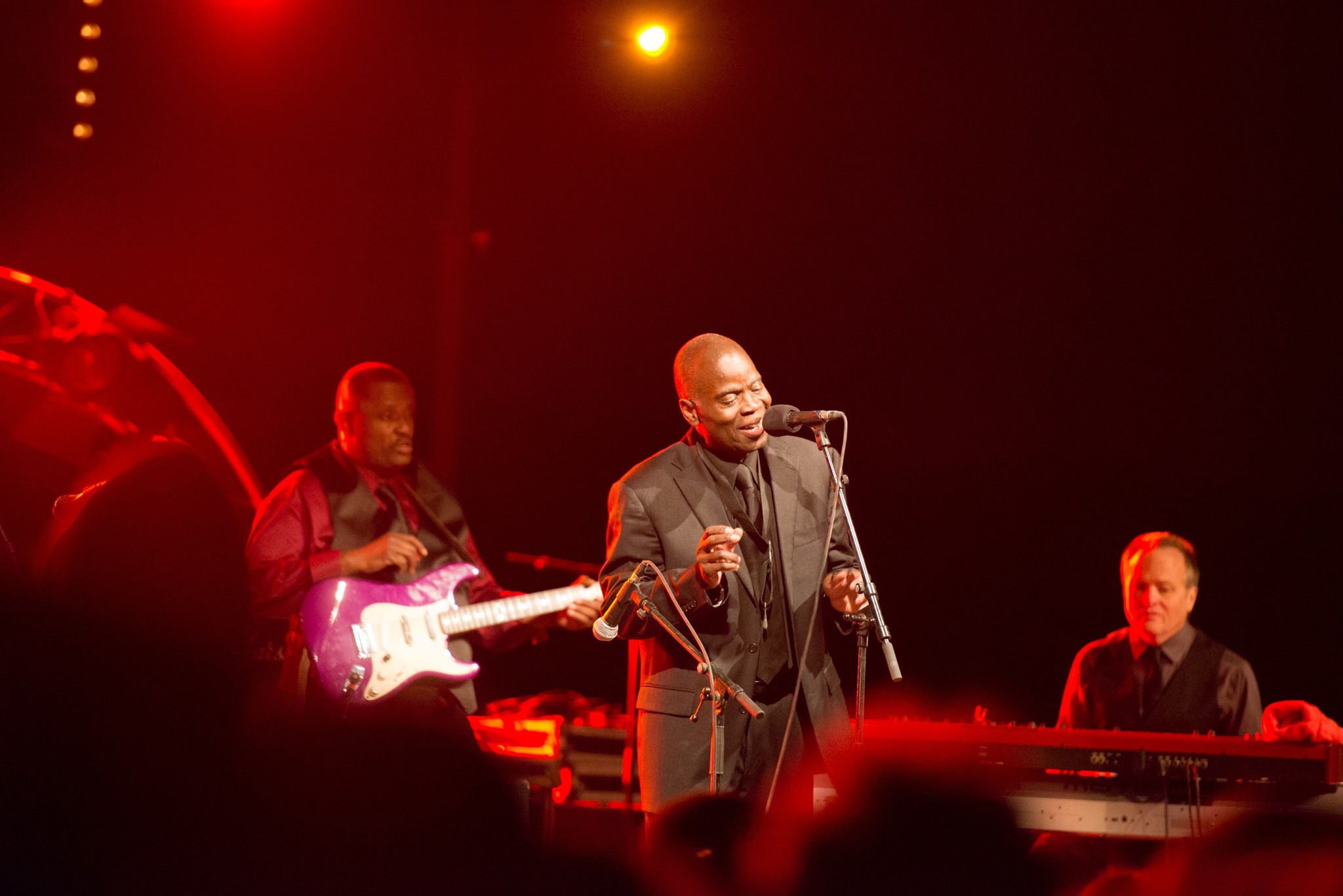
[297,560,603,709]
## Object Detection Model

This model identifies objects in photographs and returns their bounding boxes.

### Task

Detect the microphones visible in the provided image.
[593,560,648,641]
[762,405,843,437]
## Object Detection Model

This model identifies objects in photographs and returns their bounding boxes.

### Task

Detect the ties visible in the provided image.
[375,483,411,538]
[734,464,763,535]
[1142,646,1164,715]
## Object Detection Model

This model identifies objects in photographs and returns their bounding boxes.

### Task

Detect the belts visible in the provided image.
[753,679,787,697]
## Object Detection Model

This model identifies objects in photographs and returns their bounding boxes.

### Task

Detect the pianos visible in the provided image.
[844,704,1343,842]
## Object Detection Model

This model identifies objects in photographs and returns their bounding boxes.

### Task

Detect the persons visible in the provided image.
[1032,532,1260,896]
[0,450,1343,895]
[249,359,603,715]
[598,332,879,833]
